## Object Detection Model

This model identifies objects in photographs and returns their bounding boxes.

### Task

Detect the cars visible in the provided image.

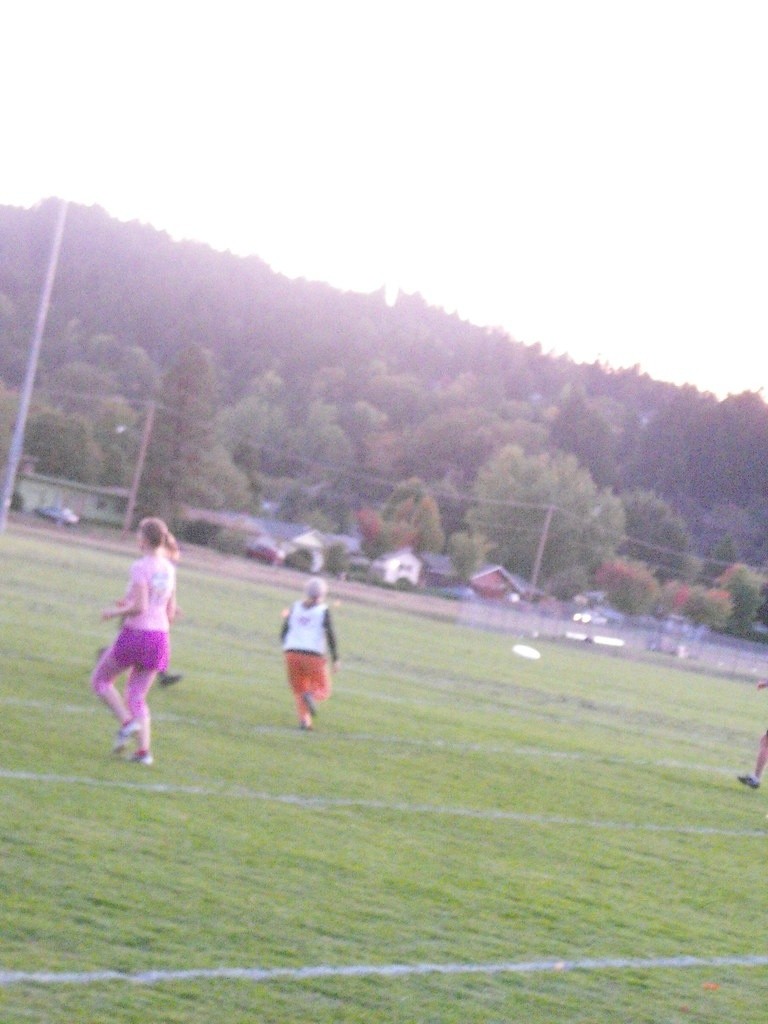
[245,535,288,565]
[34,506,80,528]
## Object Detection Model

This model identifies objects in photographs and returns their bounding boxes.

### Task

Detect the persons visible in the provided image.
[735,682,767,788]
[94,516,181,767]
[278,577,344,729]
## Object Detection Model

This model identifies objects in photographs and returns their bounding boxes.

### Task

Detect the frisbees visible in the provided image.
[513,643,542,660]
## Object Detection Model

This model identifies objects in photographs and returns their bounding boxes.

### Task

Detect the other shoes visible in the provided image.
[738,776,759,789]
[112,722,141,755]
[159,675,181,688]
[302,692,318,717]
[125,753,153,765]
[301,720,312,731]
